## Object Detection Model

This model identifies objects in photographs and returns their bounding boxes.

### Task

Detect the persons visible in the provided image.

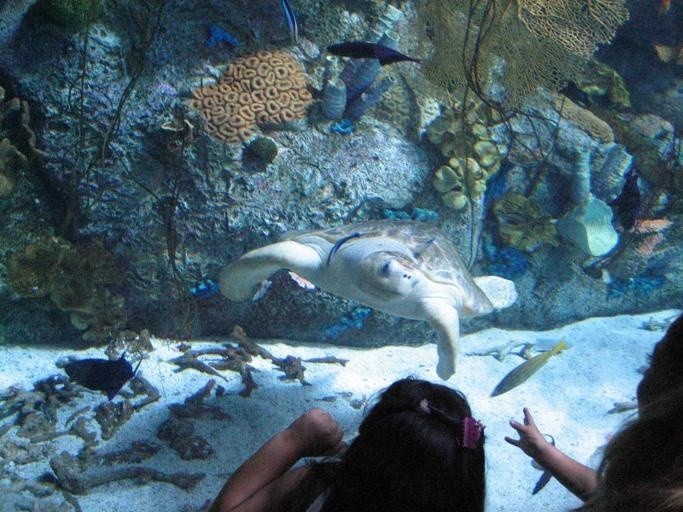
[207,375,487,512]
[503,311,683,512]
